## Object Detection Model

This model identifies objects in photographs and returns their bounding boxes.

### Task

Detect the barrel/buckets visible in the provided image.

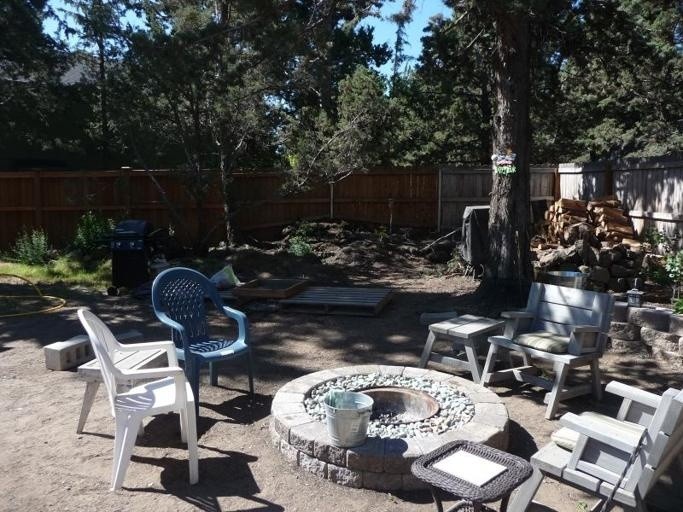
[321,390,375,449]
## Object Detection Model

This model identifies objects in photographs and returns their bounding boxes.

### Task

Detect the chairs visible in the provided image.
[510,380,683,512]
[150,266,253,416]
[480,282,614,419]
[77,309,199,493]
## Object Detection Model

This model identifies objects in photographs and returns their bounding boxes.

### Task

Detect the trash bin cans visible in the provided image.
[109,230,148,295]
[461,205,488,281]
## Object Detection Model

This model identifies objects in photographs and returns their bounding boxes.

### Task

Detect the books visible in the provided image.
[432,449,508,488]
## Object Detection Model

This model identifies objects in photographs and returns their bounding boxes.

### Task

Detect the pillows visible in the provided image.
[551,408,641,451]
[516,328,570,354]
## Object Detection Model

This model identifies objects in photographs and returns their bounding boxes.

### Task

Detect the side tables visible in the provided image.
[418,314,513,382]
[76,350,165,437]
[410,439,533,511]
